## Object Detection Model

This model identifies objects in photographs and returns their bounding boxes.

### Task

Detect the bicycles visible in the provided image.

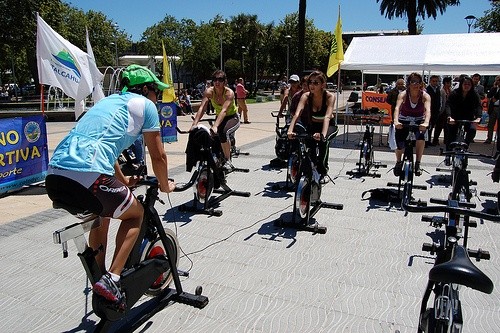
[38,103,500,333]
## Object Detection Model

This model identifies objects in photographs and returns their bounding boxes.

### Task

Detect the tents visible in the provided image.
[334,32,500,146]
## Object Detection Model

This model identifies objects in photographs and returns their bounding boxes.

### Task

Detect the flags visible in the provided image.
[161,39,177,104]
[36,12,105,101]
[75,25,106,121]
[326,5,345,78]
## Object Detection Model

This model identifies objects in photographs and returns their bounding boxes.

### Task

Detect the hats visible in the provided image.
[120,63,171,94]
[289,75,300,81]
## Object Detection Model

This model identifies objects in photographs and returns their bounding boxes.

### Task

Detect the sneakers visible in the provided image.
[394,161,402,177]
[222,161,237,171]
[415,161,423,176]
[320,176,330,183]
[93,272,128,315]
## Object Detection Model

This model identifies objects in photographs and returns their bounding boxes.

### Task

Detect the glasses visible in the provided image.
[211,76,226,82]
[307,80,321,86]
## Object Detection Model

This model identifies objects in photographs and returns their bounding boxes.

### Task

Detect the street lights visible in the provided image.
[109,21,293,84]
[463,14,476,33]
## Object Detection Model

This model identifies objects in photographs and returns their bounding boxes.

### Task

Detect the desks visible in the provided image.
[343,110,384,145]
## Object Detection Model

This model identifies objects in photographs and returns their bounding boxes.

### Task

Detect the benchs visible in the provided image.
[334,102,354,112]
[333,111,363,125]
[182,102,206,112]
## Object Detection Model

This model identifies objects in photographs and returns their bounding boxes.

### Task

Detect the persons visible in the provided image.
[236,77,251,124]
[491,153,500,183]
[224,79,229,88]
[442,76,483,167]
[44,64,177,315]
[0,80,37,98]
[276,75,302,126]
[393,72,432,176]
[286,70,339,184]
[270,73,280,96]
[172,88,196,116]
[232,79,238,106]
[290,70,324,150]
[192,70,241,170]
[205,81,214,114]
[339,78,345,94]
[360,72,500,146]
[280,76,290,111]
[192,86,204,100]
